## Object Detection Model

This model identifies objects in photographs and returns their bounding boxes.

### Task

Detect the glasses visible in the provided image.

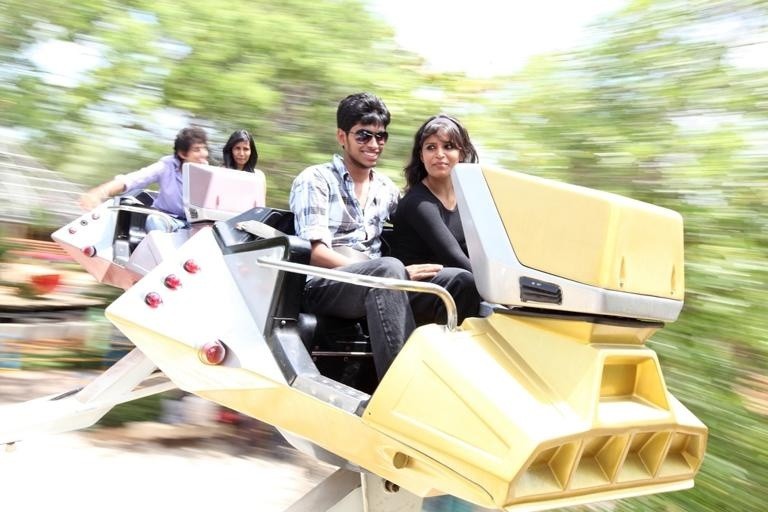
[350,128,389,145]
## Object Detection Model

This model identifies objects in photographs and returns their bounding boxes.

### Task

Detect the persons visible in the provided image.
[390,112,484,292]
[291,90,483,383]
[218,128,262,172]
[78,124,211,234]
